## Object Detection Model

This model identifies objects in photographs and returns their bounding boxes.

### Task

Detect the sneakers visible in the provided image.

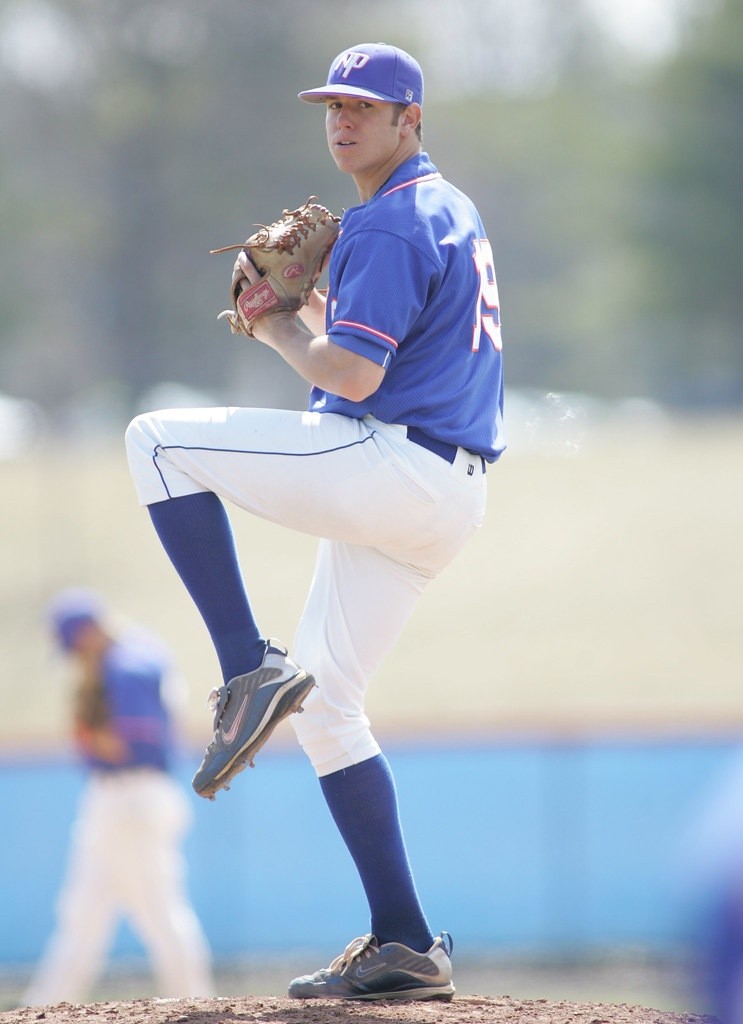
[288,932,455,1001]
[192,638,318,801]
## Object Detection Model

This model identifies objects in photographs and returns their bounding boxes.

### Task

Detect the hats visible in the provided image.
[298,43,424,109]
[56,603,93,650]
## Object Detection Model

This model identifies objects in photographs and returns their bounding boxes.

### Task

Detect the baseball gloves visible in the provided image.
[229,197,342,334]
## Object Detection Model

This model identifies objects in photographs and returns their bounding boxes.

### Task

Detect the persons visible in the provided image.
[18,609,216,1014]
[123,42,509,1005]
